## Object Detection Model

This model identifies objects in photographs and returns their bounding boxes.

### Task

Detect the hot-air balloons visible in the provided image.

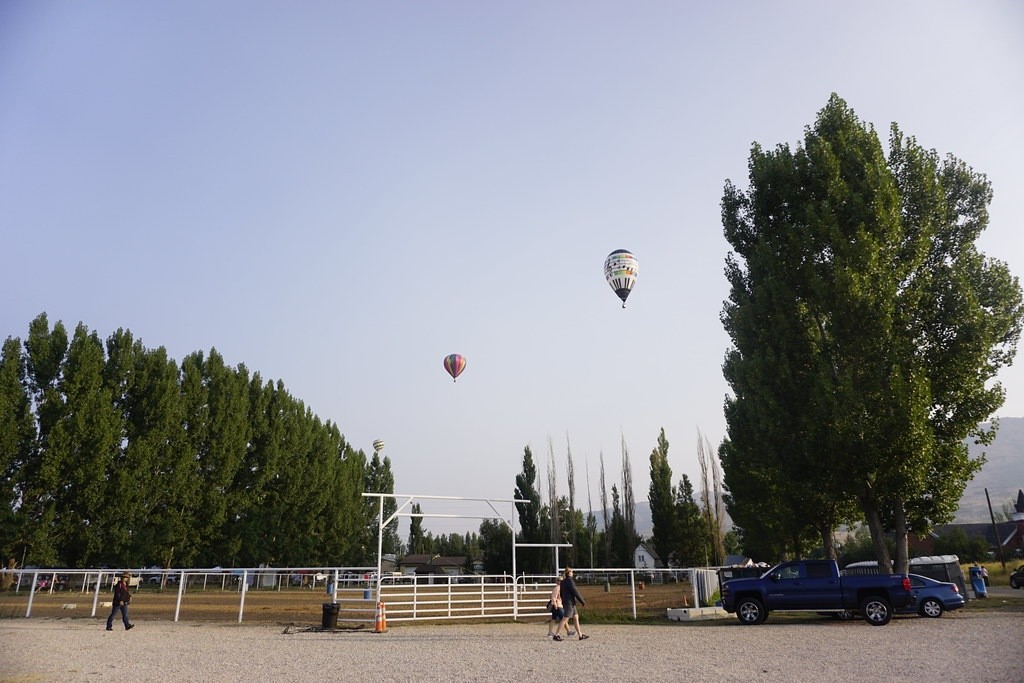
[373,439,385,452]
[604,248,640,308]
[443,353,466,382]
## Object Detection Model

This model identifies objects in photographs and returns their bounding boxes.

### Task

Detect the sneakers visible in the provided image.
[553,635,563,641]
[579,634,589,640]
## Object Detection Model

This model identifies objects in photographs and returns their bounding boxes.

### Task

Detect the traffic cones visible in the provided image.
[371,602,388,633]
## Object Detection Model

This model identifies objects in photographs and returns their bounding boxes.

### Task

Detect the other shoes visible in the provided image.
[548,633,555,637]
[126,624,134,630]
[567,631,576,636]
[106,627,113,631]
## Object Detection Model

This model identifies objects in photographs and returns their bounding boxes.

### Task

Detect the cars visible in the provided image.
[37,574,144,590]
[146,575,180,586]
[1009,564,1024,589]
[888,573,965,618]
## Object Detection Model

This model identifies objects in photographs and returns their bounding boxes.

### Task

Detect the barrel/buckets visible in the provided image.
[364,590,372,599]
[327,583,334,595]
[323,603,341,628]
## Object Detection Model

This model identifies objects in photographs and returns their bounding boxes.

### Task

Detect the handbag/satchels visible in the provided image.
[128,596,131,604]
[547,602,554,613]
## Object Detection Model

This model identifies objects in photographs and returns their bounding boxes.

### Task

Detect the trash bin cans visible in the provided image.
[715,567,774,606]
[322,602,340,629]
[969,566,988,599]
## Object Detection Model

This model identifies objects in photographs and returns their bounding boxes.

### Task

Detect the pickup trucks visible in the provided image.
[721,558,910,626]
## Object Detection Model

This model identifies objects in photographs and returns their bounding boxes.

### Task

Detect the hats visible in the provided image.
[565,568,572,576]
[557,577,563,581]
[120,572,131,577]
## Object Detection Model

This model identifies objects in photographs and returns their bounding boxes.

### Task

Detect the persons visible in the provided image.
[548,567,589,641]
[106,572,135,631]
[981,566,989,587]
